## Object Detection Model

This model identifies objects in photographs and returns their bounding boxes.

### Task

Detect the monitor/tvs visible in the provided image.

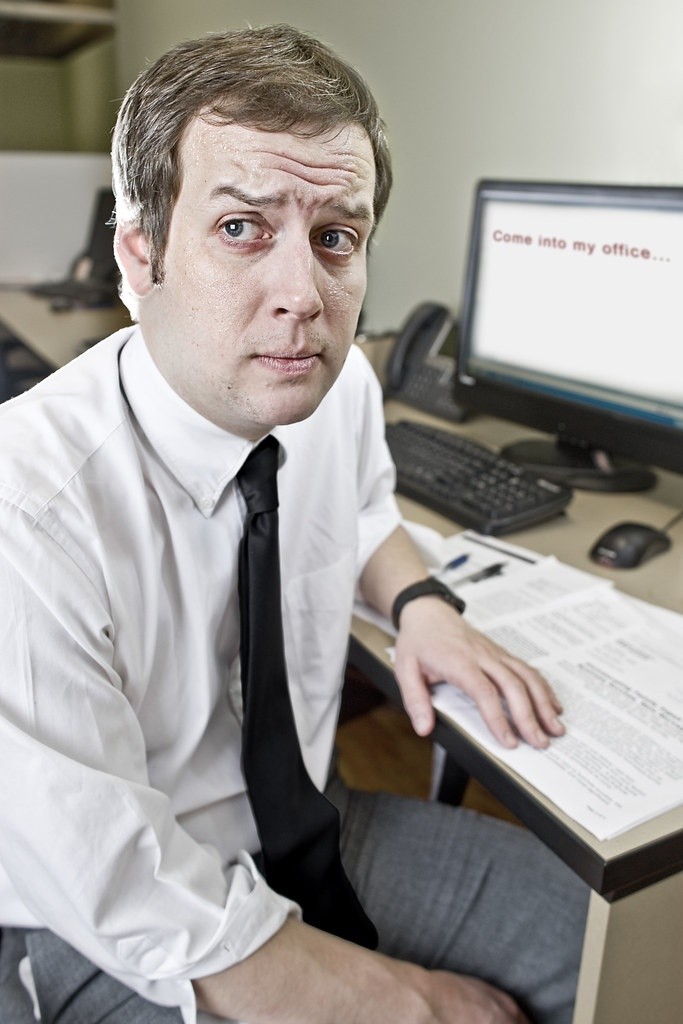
[451,177,683,493]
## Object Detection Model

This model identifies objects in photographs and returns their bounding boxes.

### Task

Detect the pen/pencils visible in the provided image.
[441,554,469,573]
[451,563,510,586]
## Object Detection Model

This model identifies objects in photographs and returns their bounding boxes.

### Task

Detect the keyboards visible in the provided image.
[386,420,572,537]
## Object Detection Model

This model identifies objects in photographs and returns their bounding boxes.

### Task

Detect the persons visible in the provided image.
[0,24,590,1024]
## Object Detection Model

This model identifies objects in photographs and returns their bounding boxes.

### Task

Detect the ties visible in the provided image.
[231,432,377,958]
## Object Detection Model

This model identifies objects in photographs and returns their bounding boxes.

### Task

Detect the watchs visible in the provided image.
[392,576,465,633]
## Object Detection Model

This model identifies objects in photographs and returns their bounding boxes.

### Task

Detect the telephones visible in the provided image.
[384,302,481,422]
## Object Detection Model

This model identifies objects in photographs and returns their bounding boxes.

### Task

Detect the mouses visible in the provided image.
[588,519,671,570]
